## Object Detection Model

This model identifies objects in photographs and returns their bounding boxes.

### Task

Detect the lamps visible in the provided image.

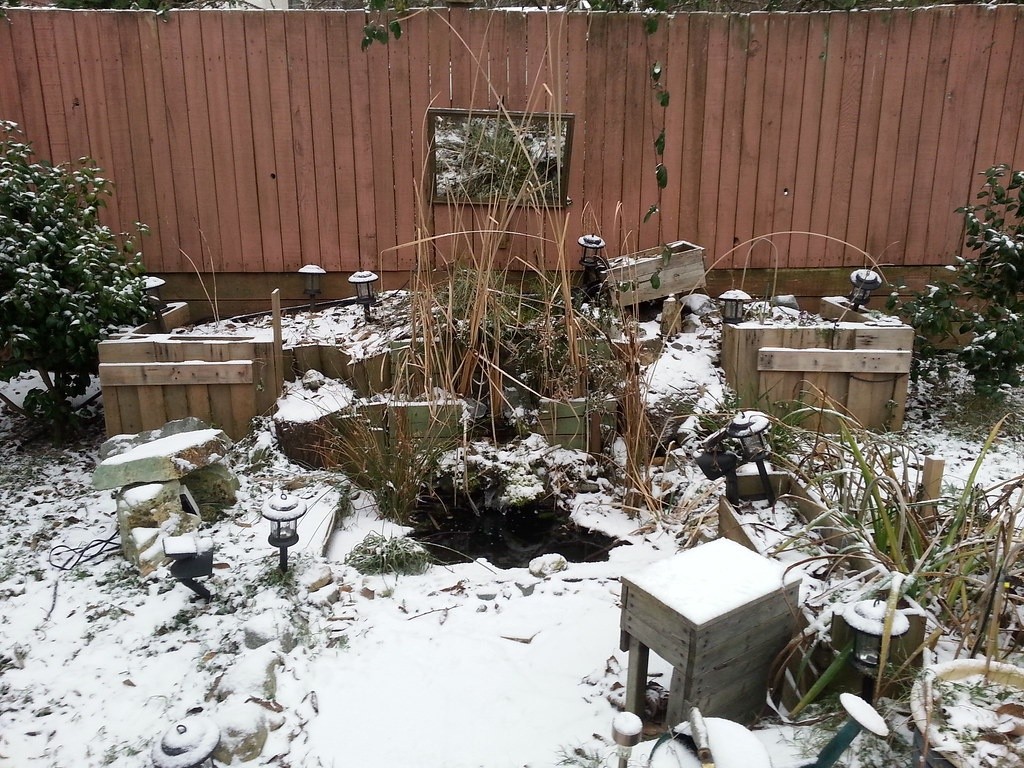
[718,289,753,324]
[150,717,222,768]
[348,268,379,303]
[261,494,308,547]
[140,277,167,309]
[726,409,773,462]
[578,234,606,267]
[849,269,882,305]
[163,535,213,600]
[612,712,643,760]
[297,263,327,295]
[842,595,911,680]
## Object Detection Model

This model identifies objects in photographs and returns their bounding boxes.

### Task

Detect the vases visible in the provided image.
[911,658,1024,768]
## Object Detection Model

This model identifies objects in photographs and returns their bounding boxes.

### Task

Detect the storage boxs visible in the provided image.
[719,471,927,702]
[598,241,706,307]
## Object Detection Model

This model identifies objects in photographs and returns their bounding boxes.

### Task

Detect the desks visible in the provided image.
[619,537,803,732]
